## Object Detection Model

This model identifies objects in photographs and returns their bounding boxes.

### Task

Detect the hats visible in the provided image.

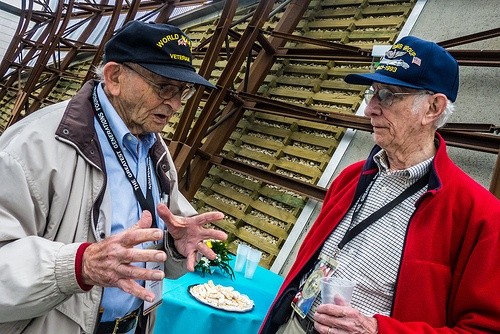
[343,35,459,102]
[103,20,219,90]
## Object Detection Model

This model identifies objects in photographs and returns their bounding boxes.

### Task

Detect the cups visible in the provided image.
[244,249,263,280]
[234,244,251,273]
[321,277,356,306]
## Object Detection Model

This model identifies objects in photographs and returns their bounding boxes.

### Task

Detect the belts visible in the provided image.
[96,308,142,334]
[294,311,320,334]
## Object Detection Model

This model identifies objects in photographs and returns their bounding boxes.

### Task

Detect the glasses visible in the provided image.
[364,87,434,106]
[113,60,197,100]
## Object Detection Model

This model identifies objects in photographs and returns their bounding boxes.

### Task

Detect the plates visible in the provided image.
[187,283,255,313]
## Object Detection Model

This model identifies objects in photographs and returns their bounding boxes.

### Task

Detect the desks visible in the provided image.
[150,262,285,334]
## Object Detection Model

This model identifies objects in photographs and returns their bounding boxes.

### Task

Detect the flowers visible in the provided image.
[195,235,236,279]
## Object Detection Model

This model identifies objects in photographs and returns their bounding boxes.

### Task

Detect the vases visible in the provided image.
[196,264,218,276]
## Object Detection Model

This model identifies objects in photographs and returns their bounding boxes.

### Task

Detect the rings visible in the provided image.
[327,327,331,334]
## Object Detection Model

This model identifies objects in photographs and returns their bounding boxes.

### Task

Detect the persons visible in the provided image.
[0,21,228,334]
[259,36,500,334]
